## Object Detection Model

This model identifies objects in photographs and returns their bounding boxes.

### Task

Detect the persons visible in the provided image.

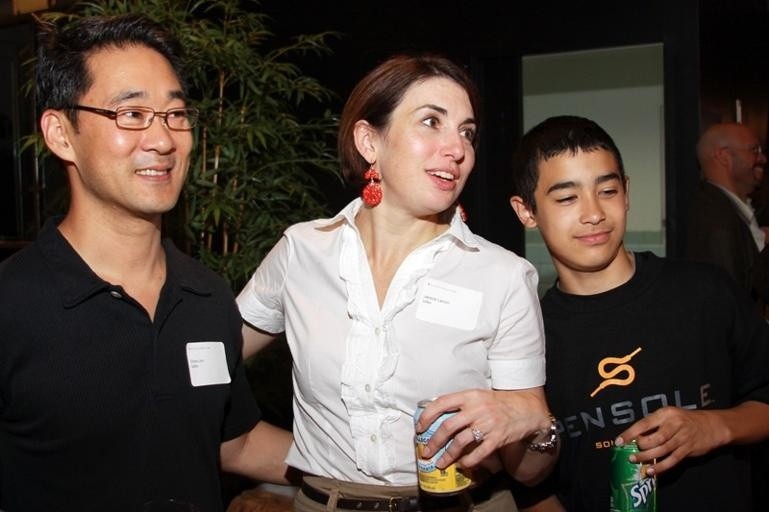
[666,119,768,280]
[0,14,293,510]
[232,56,563,510]
[509,115,768,509]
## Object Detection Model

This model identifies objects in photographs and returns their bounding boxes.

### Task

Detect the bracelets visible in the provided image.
[523,413,563,453]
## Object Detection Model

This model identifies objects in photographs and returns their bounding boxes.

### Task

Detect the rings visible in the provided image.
[469,425,484,443]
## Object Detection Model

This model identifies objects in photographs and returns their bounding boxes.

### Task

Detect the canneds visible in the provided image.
[413,398,473,497]
[609,439,657,512]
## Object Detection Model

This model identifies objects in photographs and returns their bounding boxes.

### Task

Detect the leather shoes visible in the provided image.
[300,479,512,509]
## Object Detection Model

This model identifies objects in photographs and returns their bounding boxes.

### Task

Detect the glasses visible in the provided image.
[56,104,201,134]
[722,145,765,154]
